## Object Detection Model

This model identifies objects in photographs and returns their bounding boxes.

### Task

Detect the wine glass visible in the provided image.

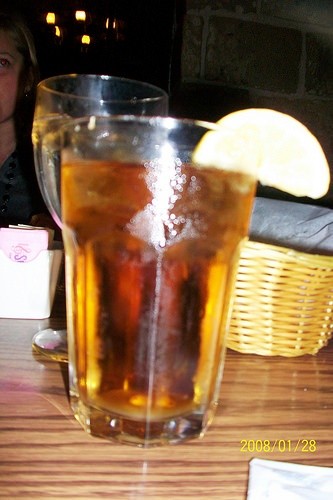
[33,73,171,366]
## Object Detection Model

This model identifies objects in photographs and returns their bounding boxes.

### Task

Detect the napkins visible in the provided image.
[1,224,63,321]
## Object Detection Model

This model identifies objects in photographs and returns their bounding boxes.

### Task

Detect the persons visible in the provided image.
[0,7,47,226]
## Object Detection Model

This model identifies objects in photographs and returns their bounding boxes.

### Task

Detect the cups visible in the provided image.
[55,116,257,446]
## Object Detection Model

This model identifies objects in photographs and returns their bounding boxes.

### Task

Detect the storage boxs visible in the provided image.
[225,238,333,357]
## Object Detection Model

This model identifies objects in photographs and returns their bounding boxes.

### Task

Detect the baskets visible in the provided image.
[226,241,333,358]
